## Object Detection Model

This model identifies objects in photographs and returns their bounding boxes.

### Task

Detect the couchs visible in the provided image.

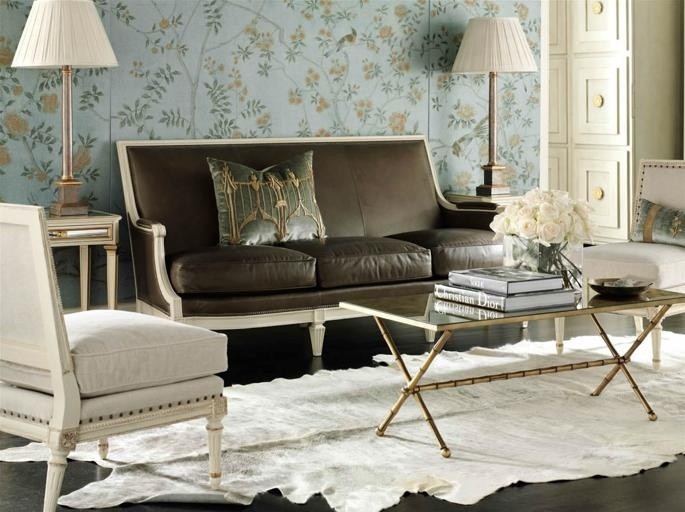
[116,135,503,356]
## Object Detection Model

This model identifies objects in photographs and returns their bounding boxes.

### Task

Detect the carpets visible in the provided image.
[0,332,685,512]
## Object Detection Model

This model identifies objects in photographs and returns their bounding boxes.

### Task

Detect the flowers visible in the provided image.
[490,187,593,248]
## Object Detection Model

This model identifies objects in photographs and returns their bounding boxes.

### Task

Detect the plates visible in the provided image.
[587,278,653,299]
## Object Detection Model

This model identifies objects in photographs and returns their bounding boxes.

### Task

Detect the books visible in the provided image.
[434,280,578,313]
[448,266,566,295]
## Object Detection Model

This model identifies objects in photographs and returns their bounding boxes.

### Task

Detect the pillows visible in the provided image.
[631,198,685,247]
[206,150,325,246]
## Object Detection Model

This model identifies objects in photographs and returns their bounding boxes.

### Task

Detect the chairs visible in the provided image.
[0,203,228,511]
[554,158,685,361]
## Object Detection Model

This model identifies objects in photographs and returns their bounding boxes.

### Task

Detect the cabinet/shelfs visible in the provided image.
[549,1,685,246]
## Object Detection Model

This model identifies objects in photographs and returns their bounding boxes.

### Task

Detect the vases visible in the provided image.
[502,235,584,305]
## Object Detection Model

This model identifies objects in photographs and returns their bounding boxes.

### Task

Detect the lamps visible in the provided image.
[12,0,119,218]
[451,16,538,196]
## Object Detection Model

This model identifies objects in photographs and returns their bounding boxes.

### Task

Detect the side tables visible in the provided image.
[447,192,520,214]
[45,209,122,310]
